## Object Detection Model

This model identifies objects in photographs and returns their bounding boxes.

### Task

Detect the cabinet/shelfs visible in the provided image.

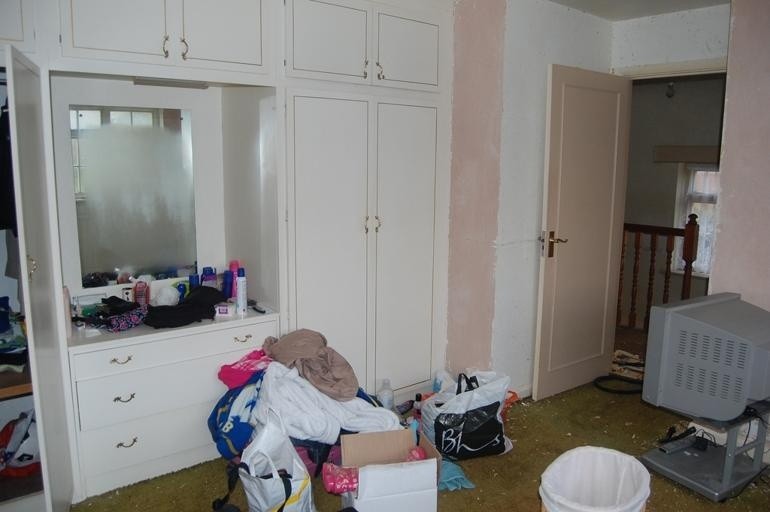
[225,88,451,416]
[285,0,456,91]
[641,399,770,502]
[48,0,285,89]
[68,314,282,505]
[0,0,48,68]
[0,360,44,504]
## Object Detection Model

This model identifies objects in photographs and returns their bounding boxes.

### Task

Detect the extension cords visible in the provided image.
[660,436,696,455]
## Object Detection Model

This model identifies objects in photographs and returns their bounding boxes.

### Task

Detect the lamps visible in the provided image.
[665,77,675,98]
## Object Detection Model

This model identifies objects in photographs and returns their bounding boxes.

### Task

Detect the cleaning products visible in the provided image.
[82,263,196,287]
[122,261,248,317]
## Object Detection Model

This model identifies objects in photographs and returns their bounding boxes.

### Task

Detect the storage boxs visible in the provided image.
[340,429,443,512]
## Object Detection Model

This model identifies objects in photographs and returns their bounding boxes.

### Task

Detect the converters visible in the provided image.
[694,437,708,451]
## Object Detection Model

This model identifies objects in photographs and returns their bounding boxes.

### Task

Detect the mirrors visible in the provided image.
[51,76,225,302]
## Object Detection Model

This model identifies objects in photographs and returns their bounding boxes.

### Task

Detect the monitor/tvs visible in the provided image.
[641,292,770,421]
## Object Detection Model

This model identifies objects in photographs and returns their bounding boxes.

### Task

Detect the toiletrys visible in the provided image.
[178,260,248,318]
[122,276,151,307]
